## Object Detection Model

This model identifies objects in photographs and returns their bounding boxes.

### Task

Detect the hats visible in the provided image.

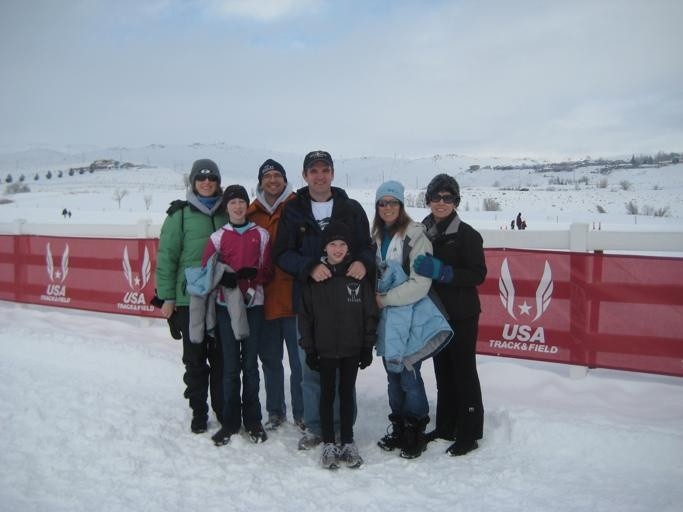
[223,185,249,208]
[303,150,334,170]
[425,173,460,204]
[190,160,221,190]
[259,159,287,183]
[376,180,404,204]
[321,222,353,252]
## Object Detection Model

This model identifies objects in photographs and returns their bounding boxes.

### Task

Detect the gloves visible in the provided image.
[219,272,238,288]
[359,348,372,369]
[239,267,257,281]
[413,253,454,284]
[306,352,319,371]
[150,296,182,339]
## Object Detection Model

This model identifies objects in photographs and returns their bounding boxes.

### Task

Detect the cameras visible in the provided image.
[244,288,256,308]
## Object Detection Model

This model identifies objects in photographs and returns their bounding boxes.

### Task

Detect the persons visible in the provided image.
[299,224,376,469]
[247,158,310,435]
[508,211,530,230]
[154,158,239,434]
[67,212,71,217]
[420,172,486,455]
[369,180,435,459]
[204,185,274,447]
[62,207,66,216]
[271,149,372,447]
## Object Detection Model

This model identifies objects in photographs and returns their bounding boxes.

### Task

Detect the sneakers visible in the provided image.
[191,414,478,469]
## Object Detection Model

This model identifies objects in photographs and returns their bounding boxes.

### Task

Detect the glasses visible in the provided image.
[429,193,455,203]
[378,200,400,208]
[195,174,217,181]
